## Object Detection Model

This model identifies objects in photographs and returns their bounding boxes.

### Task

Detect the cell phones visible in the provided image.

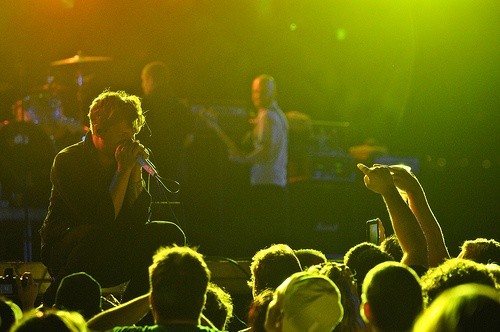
[366,220,379,245]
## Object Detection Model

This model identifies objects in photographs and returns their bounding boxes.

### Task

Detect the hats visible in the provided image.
[281,272,343,332]
[54,271,102,323]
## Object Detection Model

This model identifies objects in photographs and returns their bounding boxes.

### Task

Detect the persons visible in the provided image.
[0,59,500,332]
[40,90,186,325]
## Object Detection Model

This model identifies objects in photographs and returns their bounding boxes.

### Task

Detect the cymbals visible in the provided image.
[51,56,112,66]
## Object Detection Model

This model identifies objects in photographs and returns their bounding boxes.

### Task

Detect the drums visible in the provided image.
[12,94,55,128]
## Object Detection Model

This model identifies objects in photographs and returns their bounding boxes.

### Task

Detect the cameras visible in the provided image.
[0,275,28,296]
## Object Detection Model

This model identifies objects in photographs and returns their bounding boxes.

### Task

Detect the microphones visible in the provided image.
[135,155,157,178]
